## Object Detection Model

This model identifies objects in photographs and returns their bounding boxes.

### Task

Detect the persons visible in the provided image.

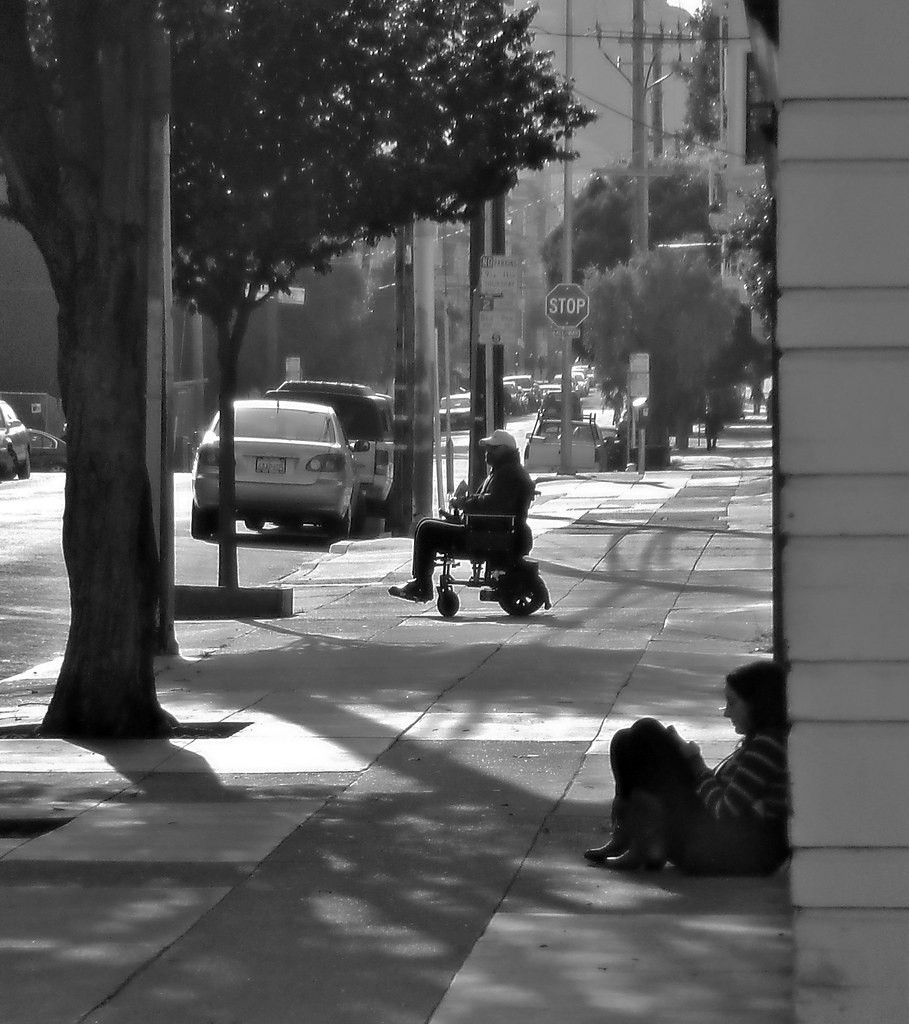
[389,430,533,599]
[583,660,789,877]
[705,413,720,452]
[765,392,772,423]
[750,382,766,415]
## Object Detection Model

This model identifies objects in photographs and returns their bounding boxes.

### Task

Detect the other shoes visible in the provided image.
[399,580,431,599]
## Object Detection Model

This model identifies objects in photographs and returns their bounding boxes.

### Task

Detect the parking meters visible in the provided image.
[638,403,650,475]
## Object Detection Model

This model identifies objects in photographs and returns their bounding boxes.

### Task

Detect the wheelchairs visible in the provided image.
[436,491,552,618]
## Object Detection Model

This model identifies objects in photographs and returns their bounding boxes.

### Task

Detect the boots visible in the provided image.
[582,795,631,863]
[606,792,662,870]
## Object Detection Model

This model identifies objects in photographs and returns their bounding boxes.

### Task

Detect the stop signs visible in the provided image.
[545,283,589,328]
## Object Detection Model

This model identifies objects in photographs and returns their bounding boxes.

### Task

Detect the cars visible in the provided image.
[618,419,670,470]
[264,379,395,525]
[538,390,584,421]
[191,400,371,544]
[439,394,470,427]
[599,427,628,472]
[502,365,595,416]
[27,428,67,472]
[0,401,31,481]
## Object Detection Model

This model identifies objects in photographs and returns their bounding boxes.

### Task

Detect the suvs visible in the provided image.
[522,413,607,473]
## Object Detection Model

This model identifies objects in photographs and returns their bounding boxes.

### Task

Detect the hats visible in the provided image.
[479,430,515,451]
